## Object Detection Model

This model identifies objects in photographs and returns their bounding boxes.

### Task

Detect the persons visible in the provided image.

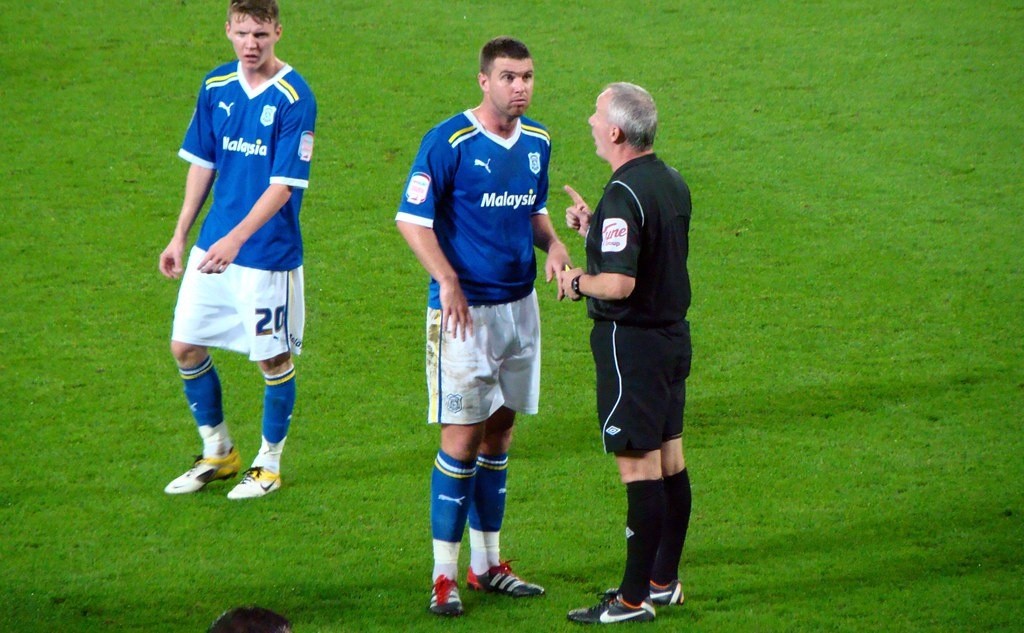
[394,35,574,618]
[560,82,692,624]
[155,0,316,498]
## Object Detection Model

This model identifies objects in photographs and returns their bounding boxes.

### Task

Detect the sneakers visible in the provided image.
[430,574,464,616]
[567,591,657,624]
[164,446,241,494]
[227,466,281,500]
[466,560,545,597]
[604,579,684,606]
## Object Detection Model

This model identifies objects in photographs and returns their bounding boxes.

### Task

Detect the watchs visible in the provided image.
[571,275,585,297]
